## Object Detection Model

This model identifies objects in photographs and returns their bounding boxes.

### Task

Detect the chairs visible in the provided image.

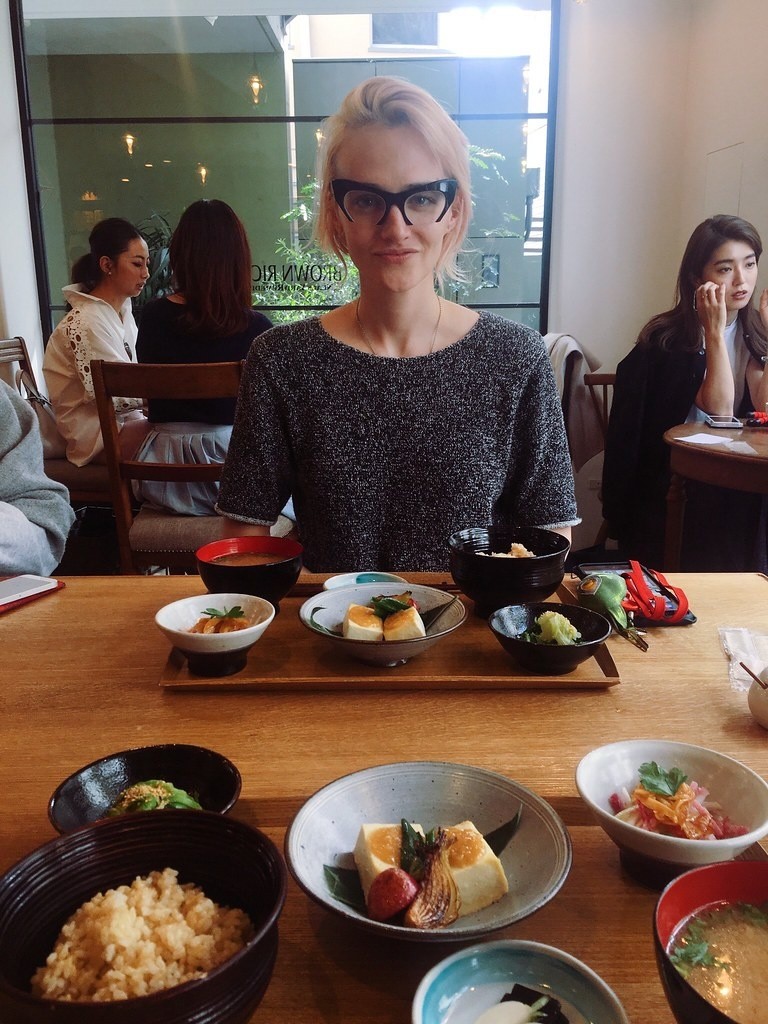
[543,334,623,563]
[89,354,247,576]
[0,336,115,503]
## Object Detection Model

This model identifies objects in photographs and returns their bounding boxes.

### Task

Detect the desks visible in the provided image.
[664,417,768,576]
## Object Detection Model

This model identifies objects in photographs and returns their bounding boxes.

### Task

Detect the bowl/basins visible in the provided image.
[652,860,768,1024]
[322,571,409,591]
[298,582,469,667]
[575,740,768,870]
[0,809,289,1024]
[448,525,572,620]
[283,760,574,943]
[487,602,613,676]
[194,536,306,616]
[411,940,630,1024]
[155,593,276,678]
[48,744,243,837]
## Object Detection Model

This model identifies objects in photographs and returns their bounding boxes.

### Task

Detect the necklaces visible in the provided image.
[356,297,443,403]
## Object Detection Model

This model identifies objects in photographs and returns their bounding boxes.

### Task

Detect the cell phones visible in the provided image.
[577,562,694,625]
[706,416,744,428]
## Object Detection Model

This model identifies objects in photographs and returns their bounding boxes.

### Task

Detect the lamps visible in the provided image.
[243,54,268,110]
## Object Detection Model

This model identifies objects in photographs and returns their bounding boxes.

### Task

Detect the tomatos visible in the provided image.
[367,867,416,921]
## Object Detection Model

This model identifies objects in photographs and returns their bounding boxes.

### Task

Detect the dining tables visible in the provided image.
[0,574,768,1024]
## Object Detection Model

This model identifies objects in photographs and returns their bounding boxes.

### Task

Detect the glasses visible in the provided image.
[326,178,457,227]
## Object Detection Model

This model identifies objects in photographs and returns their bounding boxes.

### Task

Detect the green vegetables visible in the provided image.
[400,818,439,883]
[667,900,768,984]
[103,781,206,817]
[638,761,690,796]
[201,604,245,620]
[369,596,409,618]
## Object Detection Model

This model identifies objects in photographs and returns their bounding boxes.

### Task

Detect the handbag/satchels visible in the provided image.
[15,369,68,459]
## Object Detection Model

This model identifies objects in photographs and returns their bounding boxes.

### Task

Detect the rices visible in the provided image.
[30,868,257,1003]
[476,543,536,557]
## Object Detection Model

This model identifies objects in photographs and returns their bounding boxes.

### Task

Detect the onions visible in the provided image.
[405,831,462,928]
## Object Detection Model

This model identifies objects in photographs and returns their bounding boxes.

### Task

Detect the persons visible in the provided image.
[126,198,297,522]
[214,76,582,573]
[601,213,768,572]
[42,218,152,468]
[0,378,76,577]
